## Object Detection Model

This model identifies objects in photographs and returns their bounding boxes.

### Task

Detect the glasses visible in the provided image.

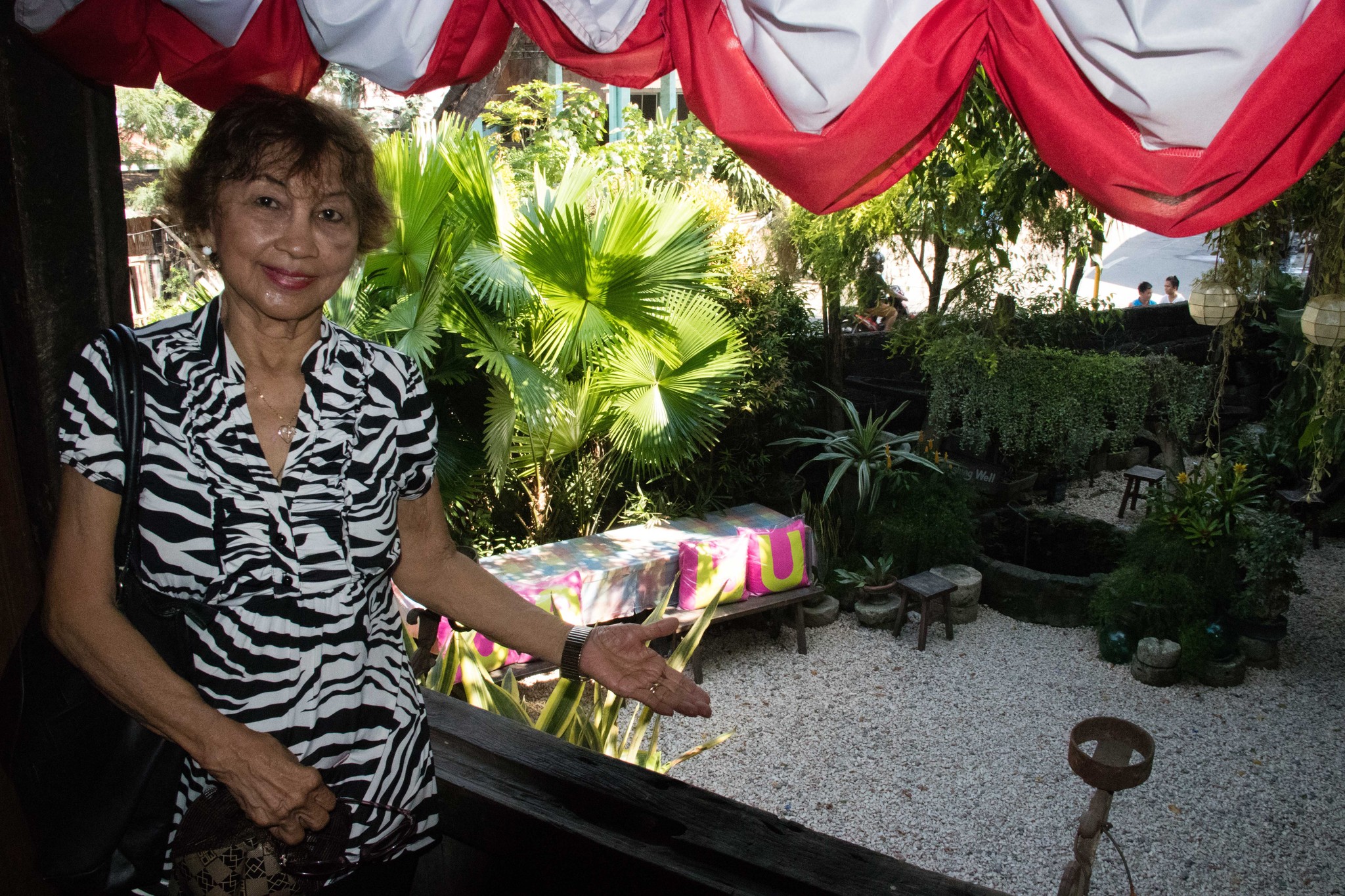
[274,797,417,878]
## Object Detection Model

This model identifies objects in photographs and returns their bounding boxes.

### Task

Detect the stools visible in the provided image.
[893,570,958,651]
[1118,465,1166,519]
[1275,490,1326,549]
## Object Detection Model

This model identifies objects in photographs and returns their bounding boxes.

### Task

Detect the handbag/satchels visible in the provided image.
[0,317,195,896]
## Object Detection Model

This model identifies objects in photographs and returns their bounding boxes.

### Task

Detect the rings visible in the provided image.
[650,683,662,694]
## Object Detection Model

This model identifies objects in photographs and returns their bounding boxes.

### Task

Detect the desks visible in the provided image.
[392,501,818,686]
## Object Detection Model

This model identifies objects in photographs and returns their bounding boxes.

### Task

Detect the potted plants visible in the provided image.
[1088,513,1311,681]
[733,278,982,605]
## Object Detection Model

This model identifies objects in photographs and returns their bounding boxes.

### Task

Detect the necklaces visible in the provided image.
[246,375,298,443]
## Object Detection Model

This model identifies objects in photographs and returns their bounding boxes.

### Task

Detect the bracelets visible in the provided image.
[560,625,593,683]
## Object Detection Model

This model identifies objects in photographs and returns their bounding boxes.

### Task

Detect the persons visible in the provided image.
[1158,275,1186,304]
[47,83,712,896]
[1128,282,1157,307]
[858,252,908,333]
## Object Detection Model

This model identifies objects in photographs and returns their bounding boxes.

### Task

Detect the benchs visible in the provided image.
[451,584,826,685]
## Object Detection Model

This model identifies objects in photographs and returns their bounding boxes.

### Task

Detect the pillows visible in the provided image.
[734,513,811,596]
[675,532,749,611]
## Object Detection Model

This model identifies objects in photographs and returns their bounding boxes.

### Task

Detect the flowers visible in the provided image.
[1141,459,1270,547]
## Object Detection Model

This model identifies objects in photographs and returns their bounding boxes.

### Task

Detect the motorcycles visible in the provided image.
[840,287,913,334]
